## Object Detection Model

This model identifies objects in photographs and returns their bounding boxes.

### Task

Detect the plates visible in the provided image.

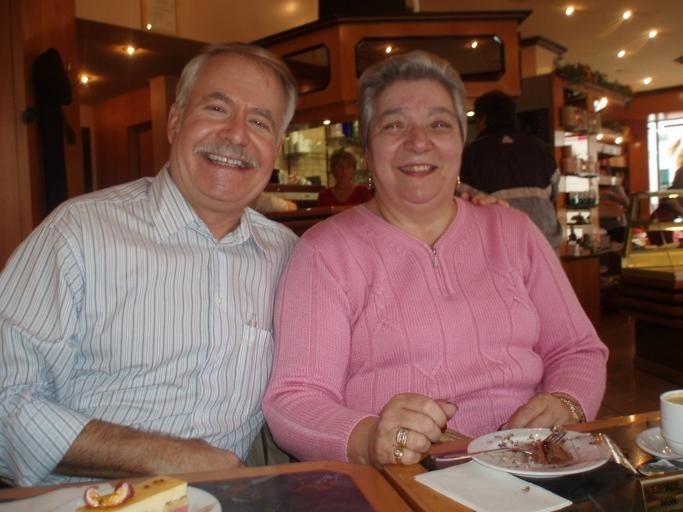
[635,426,683,466]
[468,427,616,478]
[56,484,221,512]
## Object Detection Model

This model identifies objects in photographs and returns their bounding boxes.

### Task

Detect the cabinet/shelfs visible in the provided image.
[555,103,633,258]
[619,186,682,370]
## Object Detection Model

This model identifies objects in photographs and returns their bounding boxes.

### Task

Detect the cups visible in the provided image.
[658,389,683,455]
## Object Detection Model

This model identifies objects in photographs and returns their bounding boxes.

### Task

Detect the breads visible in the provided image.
[75,474,188,512]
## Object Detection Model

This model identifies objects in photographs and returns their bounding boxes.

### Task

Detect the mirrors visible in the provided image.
[354,36,507,82]
[282,44,329,95]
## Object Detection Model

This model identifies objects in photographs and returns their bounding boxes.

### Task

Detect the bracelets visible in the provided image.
[558,398,585,423]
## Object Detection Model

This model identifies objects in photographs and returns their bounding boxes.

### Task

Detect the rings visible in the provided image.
[394,448,402,462]
[396,430,408,446]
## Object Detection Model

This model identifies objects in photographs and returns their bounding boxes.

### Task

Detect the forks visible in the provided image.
[431,429,566,464]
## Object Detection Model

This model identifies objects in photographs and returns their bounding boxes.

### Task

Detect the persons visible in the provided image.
[649,168,683,244]
[317,150,371,205]
[252,193,297,214]
[462,91,564,250]
[0,42,508,489]
[604,170,630,314]
[264,48,611,467]
